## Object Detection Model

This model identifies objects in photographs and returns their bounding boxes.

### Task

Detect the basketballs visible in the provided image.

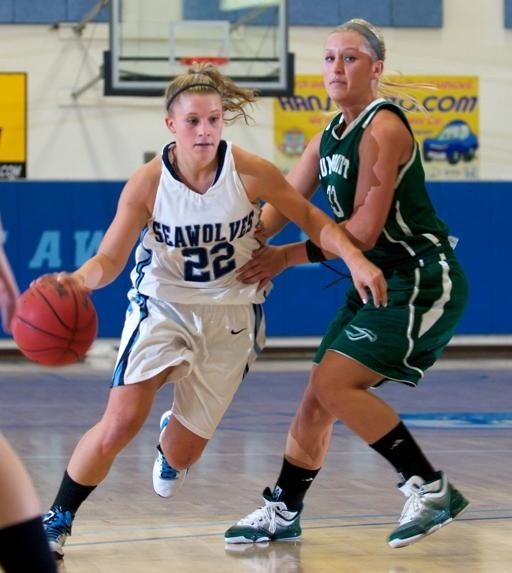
[9,275,97,366]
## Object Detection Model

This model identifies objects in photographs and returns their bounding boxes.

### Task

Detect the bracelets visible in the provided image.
[305,238,326,265]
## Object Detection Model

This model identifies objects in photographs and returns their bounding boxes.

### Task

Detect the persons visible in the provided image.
[0,244,58,572]
[26,59,390,556]
[220,18,469,550]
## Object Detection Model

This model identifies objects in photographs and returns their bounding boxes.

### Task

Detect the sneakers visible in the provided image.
[152,410,191,499]
[387,470,471,549]
[224,487,304,544]
[40,504,72,560]
